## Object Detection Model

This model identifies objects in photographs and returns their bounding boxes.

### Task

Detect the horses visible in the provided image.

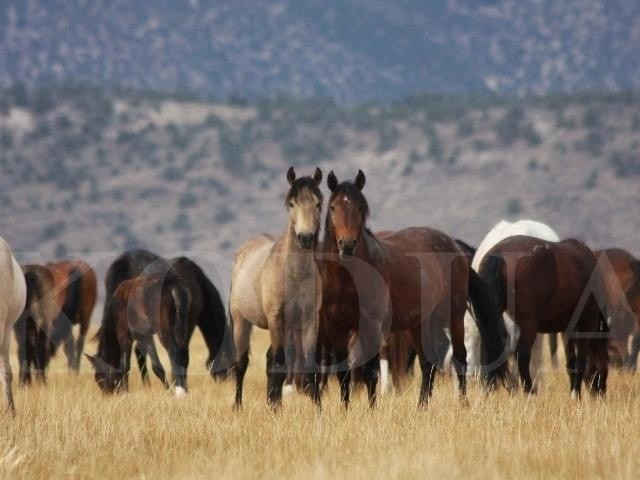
[228,164,325,419]
[45,259,98,376]
[11,264,55,387]
[83,248,234,399]
[0,237,27,418]
[285,218,639,413]
